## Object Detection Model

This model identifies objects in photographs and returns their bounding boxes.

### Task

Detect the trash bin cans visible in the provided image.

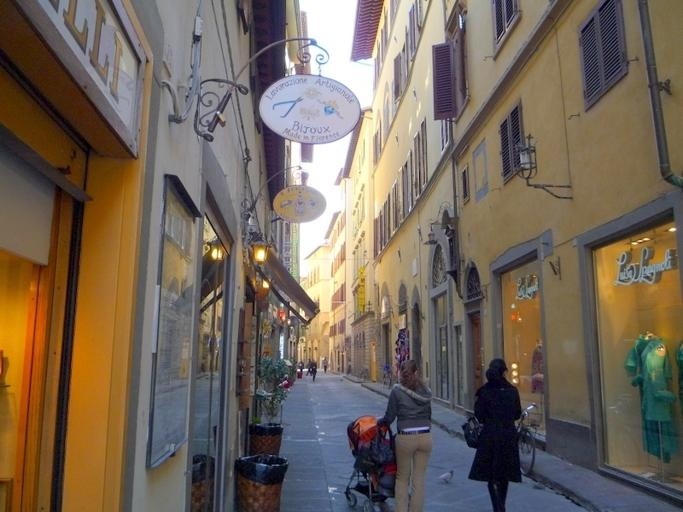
[190,454,215,512]
[297,372,302,378]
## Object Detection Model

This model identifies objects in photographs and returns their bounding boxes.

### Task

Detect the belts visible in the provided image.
[397,428,432,435]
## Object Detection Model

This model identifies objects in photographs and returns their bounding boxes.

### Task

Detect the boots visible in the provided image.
[488,482,509,512]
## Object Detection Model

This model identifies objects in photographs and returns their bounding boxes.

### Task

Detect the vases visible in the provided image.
[264,382,274,393]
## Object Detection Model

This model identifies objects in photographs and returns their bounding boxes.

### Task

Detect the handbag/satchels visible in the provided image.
[369,443,395,463]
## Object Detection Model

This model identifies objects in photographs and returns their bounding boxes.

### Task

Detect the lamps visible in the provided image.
[512,134,573,199]
[248,231,272,267]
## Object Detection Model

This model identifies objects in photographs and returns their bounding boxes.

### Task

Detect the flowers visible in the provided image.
[256,354,298,423]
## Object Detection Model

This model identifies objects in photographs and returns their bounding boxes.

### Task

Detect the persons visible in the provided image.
[297,359,304,372]
[322,357,328,374]
[528,338,546,396]
[467,358,522,511]
[624,330,678,464]
[374,358,434,511]
[305,358,311,376]
[308,359,316,381]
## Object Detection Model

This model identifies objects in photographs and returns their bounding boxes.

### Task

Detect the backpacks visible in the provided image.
[461,423,481,449]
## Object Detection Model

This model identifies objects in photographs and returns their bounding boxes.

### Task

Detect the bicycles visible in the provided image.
[380,365,392,391]
[513,401,539,478]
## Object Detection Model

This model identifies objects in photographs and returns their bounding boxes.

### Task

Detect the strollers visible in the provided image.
[340,414,399,512]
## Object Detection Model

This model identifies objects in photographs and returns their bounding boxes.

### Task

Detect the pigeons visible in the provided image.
[436,470,454,483]
